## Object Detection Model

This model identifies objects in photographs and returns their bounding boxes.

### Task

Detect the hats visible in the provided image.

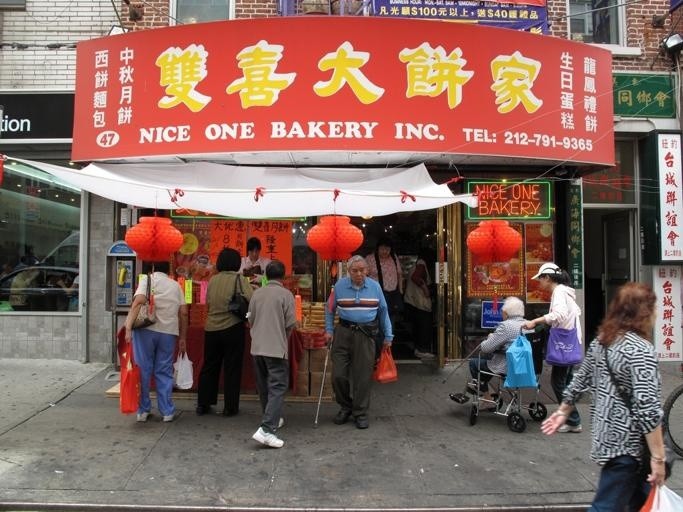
[531,262,562,281]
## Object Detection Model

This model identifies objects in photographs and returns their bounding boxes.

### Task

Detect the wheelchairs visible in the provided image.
[448,323,547,433]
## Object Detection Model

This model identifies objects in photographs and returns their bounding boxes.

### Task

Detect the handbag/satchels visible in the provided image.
[229,292,249,322]
[121,342,141,414]
[503,336,538,388]
[640,422,675,483]
[357,320,379,337]
[124,303,156,329]
[545,328,582,366]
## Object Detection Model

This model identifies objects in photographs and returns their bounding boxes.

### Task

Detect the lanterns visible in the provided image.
[306,216,364,261]
[466,220,522,262]
[125,215,184,261]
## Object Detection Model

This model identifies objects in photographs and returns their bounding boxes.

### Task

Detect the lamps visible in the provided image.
[107,24,127,36]
[661,30,683,61]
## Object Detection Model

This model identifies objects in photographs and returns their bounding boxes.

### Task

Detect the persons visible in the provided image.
[196,247,254,417]
[237,237,270,280]
[365,237,404,369]
[523,262,582,433]
[539,282,667,512]
[403,247,438,359]
[0,258,79,311]
[126,262,189,422]
[324,254,395,429]
[247,261,296,448]
[469,297,536,412]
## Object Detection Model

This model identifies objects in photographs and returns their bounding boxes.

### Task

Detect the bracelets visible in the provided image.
[556,411,568,416]
[651,456,667,464]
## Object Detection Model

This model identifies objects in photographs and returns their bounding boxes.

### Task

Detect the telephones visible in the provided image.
[116,260,133,306]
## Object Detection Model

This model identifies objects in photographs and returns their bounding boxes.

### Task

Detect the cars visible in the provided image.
[0,264,79,312]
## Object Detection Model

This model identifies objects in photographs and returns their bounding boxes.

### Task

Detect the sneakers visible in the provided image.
[136,412,149,422]
[466,382,488,397]
[163,415,174,422]
[252,426,284,448]
[355,415,369,428]
[273,417,284,432]
[196,406,211,416]
[333,408,352,424]
[414,349,435,358]
[557,424,583,433]
